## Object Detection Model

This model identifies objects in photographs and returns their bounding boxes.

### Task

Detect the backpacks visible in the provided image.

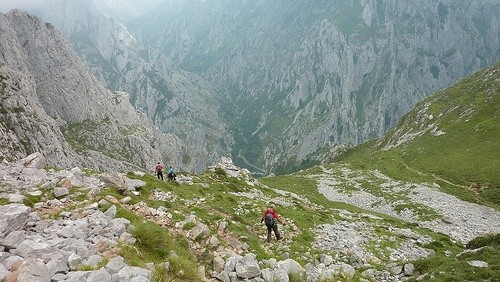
[265,210,275,229]
[168,169,174,178]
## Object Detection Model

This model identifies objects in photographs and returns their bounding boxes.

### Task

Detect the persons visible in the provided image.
[167,166,176,182]
[155,162,164,181]
[261,204,286,243]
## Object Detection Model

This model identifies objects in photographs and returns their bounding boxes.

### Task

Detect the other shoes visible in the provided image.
[277,237,284,241]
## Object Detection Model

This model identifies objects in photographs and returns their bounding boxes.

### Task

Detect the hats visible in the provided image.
[269,204,276,209]
